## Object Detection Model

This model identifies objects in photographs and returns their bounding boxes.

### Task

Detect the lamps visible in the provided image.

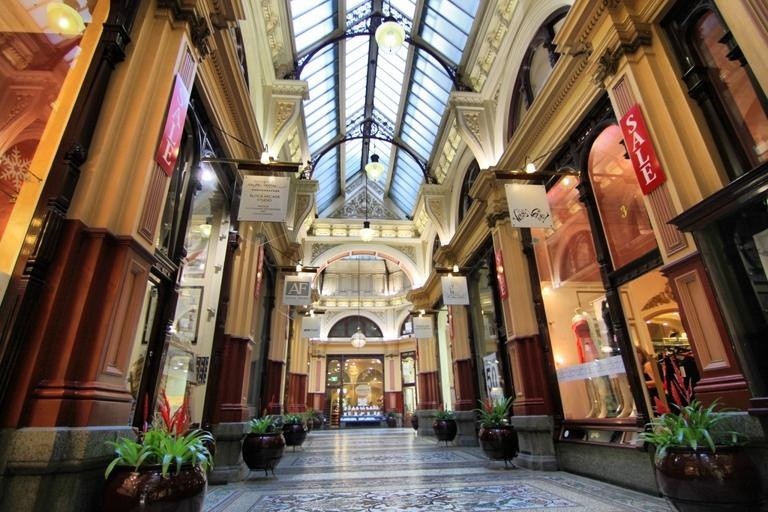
[366,136,385,179]
[357,170,376,243]
[259,143,271,165]
[349,257,367,350]
[370,0,407,53]
[48,2,87,35]
[521,155,537,174]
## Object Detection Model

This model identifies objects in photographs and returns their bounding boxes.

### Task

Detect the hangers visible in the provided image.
[654,346,691,362]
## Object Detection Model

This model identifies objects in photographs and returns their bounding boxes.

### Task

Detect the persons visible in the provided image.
[634,345,663,417]
[595,294,636,419]
[570,305,608,417]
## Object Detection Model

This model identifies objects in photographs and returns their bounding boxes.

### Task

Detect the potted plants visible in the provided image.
[432,407,458,442]
[386,412,401,427]
[409,409,419,431]
[307,407,314,433]
[283,414,305,447]
[243,415,285,472]
[636,399,761,512]
[474,398,521,462]
[101,422,215,512]
[315,411,324,429]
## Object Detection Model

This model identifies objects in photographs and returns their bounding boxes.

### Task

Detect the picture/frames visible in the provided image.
[173,286,205,345]
